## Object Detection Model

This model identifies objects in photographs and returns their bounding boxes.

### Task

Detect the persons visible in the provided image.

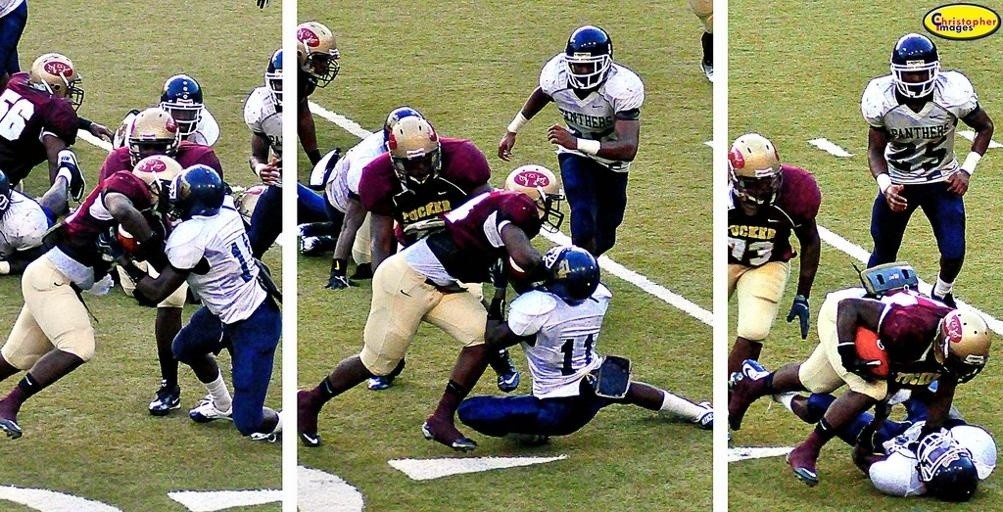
[298,1,712,452]
[0,0,282,445]
[725,32,997,503]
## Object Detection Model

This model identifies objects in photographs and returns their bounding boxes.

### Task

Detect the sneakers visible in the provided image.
[250,410,282,443]
[148,379,181,415]
[189,397,233,423]
[701,59,713,82]
[931,283,956,308]
[422,421,476,452]
[490,349,519,391]
[729,372,743,430]
[298,390,320,446]
[300,236,323,256]
[0,417,22,439]
[367,358,406,389]
[57,150,86,202]
[698,400,713,430]
[518,433,548,447]
[741,359,786,412]
[785,452,819,486]
[297,222,312,237]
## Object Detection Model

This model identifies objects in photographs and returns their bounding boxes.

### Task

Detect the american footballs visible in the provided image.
[503,250,535,281]
[116,223,140,255]
[856,324,892,380]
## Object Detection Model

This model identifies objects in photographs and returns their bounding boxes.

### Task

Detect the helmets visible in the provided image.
[31,53,84,112]
[565,25,612,90]
[385,116,442,185]
[128,108,180,167]
[505,164,564,234]
[132,155,182,202]
[917,432,978,501]
[160,75,203,136]
[0,169,10,219]
[384,106,424,150]
[265,49,283,107]
[934,309,991,383]
[542,244,600,306]
[728,133,784,209]
[170,164,225,218]
[297,22,341,88]
[890,33,939,99]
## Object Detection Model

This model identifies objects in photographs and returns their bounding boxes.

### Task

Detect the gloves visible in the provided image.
[787,295,810,338]
[325,271,359,289]
[837,342,880,382]
[349,263,373,279]
[96,226,124,260]
[489,258,507,290]
